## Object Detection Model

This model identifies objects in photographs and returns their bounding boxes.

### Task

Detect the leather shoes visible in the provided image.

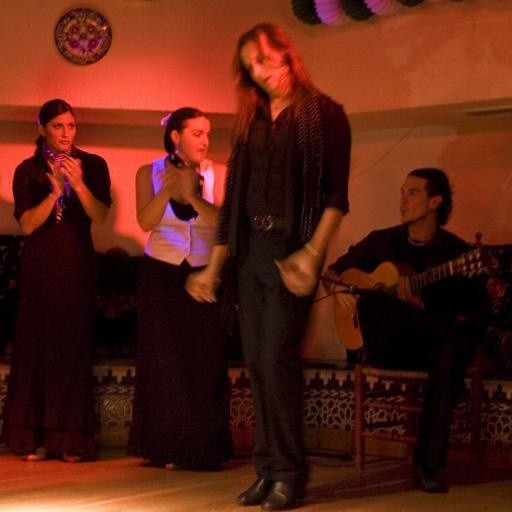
[260,474,306,511]
[413,466,450,493]
[490,328,512,364]
[236,474,272,506]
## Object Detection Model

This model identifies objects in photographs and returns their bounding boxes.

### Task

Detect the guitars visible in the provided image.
[333,233,498,350]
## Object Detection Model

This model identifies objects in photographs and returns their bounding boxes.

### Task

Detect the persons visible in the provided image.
[133,103,229,473]
[10,96,113,466]
[321,165,506,497]
[182,17,355,512]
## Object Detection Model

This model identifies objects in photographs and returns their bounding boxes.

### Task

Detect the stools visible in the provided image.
[353,363,482,483]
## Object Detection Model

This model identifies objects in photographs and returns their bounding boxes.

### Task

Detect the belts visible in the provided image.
[240,216,288,231]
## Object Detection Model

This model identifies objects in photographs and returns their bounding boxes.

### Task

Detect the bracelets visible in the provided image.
[303,241,325,261]
